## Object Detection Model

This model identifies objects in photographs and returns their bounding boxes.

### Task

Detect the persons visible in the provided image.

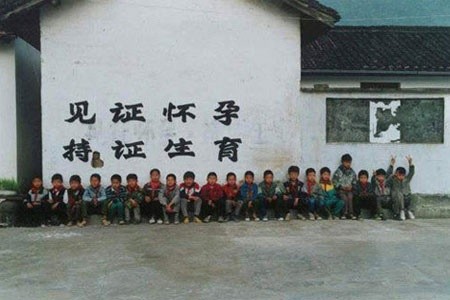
[22,153,415,228]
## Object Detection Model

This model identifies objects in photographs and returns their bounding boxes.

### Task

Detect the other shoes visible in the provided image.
[41,220,87,228]
[374,214,384,221]
[245,213,305,221]
[394,210,416,220]
[309,213,364,220]
[101,218,179,225]
[183,216,239,224]
